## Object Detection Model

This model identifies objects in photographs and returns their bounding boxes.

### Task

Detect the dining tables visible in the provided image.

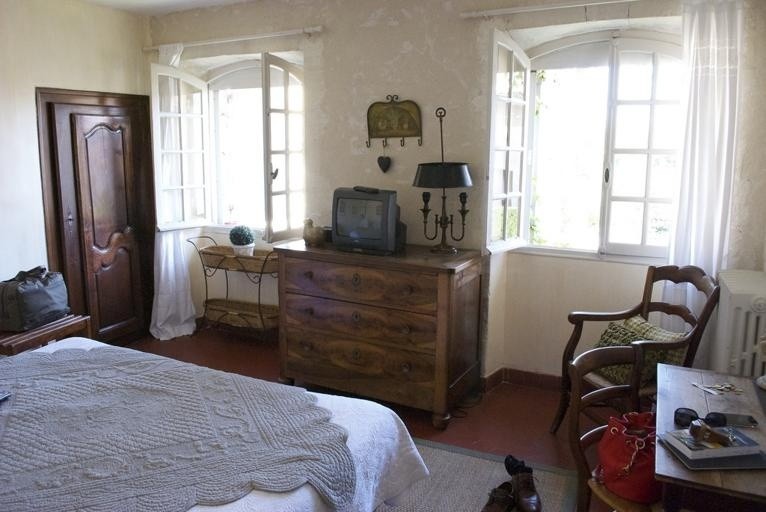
[229,226,255,256]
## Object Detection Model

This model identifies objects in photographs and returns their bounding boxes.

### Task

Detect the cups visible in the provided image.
[674,408,726,428]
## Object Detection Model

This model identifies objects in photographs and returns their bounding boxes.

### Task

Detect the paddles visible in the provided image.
[389,437,578,512]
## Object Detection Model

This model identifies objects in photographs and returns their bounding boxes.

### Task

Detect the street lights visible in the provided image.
[595,412,663,504]
[0,265,71,332]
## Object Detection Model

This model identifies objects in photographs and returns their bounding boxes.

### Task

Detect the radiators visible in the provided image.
[0,390,11,402]
[353,186,379,194]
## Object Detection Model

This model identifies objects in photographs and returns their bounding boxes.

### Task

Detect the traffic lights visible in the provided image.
[624,315,689,367]
[590,321,665,390]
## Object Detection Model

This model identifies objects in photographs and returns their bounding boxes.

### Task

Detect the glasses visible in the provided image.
[412,162,474,253]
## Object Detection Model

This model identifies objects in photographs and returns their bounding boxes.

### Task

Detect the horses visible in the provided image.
[721,413,758,428]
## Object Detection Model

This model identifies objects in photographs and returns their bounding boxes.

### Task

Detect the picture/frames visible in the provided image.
[654,362,766,512]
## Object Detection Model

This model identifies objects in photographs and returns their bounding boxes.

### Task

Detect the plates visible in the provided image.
[187,236,278,350]
[0,314,93,356]
[275,238,491,430]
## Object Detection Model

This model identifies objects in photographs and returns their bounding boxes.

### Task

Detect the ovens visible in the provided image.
[0,336,429,512]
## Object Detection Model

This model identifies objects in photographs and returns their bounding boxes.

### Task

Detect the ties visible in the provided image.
[332,187,407,256]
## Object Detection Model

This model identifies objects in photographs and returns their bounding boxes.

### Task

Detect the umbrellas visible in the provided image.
[512,473,541,512]
[481,481,514,512]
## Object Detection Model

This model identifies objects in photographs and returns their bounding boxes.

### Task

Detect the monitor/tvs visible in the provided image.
[659,424,766,471]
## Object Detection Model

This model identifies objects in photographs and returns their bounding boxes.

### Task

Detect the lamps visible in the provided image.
[550,265,720,434]
[568,346,674,512]
